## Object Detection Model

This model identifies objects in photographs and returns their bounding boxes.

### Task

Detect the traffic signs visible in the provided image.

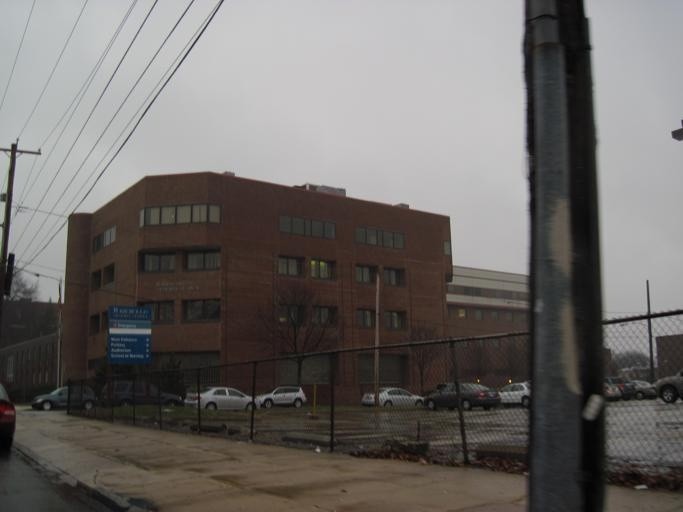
[106,304,152,366]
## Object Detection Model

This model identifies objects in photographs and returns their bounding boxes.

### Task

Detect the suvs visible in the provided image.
[99,377,186,410]
[655,367,683,405]
[252,384,309,410]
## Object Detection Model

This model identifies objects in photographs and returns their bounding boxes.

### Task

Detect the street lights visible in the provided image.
[619,322,661,336]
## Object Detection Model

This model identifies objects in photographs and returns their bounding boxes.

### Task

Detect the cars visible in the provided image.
[0,380,16,455]
[29,383,98,412]
[422,381,500,412]
[604,376,658,404]
[359,384,426,409]
[493,381,530,408]
[180,384,265,412]
